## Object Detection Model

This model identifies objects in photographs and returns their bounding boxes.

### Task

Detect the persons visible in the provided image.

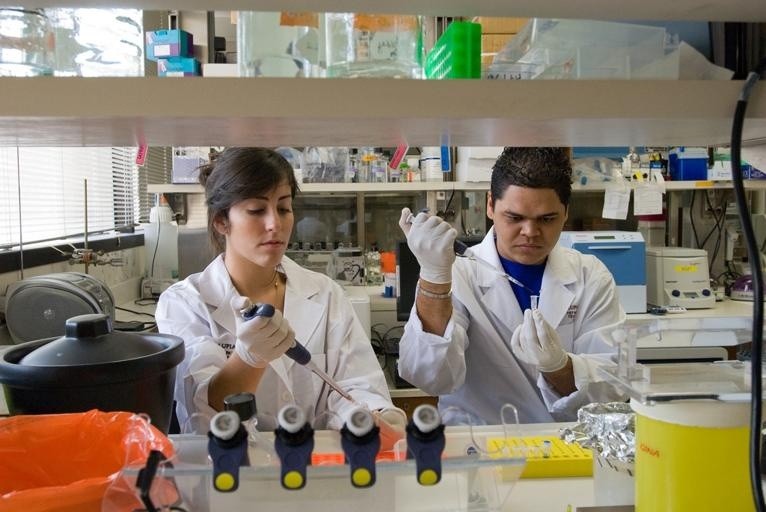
[153,148,410,453]
[397,145,630,425]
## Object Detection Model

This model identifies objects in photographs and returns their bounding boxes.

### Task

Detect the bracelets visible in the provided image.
[418,286,452,299]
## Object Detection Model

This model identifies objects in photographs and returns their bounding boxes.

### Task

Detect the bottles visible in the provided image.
[501,438,553,460]
[221,390,275,469]
[345,147,445,184]
[0,6,56,75]
[357,402,404,442]
[285,239,354,267]
[365,239,382,287]
[530,294,538,310]
[616,326,638,379]
[626,147,642,169]
